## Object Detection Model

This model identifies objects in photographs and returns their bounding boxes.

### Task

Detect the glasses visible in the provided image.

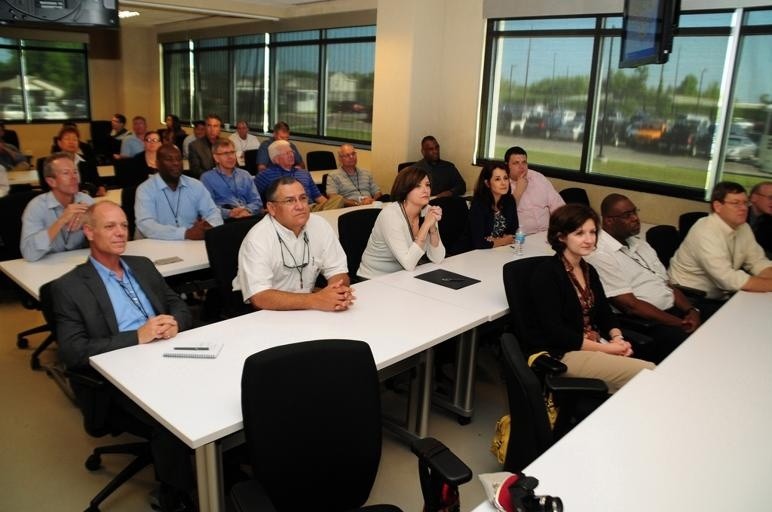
[607,207,640,219]
[281,243,309,268]
[215,150,237,157]
[144,138,160,143]
[723,199,753,208]
[270,196,309,206]
[754,192,772,199]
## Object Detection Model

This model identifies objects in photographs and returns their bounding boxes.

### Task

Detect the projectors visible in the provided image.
[0,0,119,27]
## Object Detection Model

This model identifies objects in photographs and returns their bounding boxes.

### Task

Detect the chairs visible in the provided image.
[37,275,155,511]
[499,331,613,476]
[645,224,708,323]
[226,338,474,512]
[678,212,709,242]
[0,183,59,369]
[502,253,654,383]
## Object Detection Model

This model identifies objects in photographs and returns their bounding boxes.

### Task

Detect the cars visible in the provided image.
[333,99,365,112]
[496,102,772,172]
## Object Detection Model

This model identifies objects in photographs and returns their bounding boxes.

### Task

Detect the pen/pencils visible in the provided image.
[442,277,466,282]
[174,347,210,350]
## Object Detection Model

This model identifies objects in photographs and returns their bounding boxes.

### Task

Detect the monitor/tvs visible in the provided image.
[619,0,681,68]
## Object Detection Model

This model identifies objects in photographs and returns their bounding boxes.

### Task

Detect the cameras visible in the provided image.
[508,477,563,512]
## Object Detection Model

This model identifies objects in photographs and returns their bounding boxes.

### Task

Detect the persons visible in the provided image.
[523,204,656,393]
[356,168,447,397]
[200,138,263,219]
[19,152,95,263]
[746,182,772,261]
[44,200,240,512]
[505,147,567,238]
[585,193,720,350]
[325,144,383,206]
[669,181,772,323]
[232,176,357,312]
[467,160,519,249]
[256,140,343,212]
[132,143,224,239]
[413,136,466,203]
[1,111,306,199]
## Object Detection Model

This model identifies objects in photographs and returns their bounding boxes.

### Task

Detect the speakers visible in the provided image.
[88,27,120,59]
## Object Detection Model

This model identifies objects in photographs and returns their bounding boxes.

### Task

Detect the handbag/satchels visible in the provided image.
[491,396,557,466]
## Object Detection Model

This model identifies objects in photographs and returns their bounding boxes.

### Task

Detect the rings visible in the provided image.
[167,335,170,338]
[75,216,81,222]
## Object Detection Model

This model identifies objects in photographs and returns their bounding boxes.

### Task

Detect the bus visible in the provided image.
[2,99,87,119]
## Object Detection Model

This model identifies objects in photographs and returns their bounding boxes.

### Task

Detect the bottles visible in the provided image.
[513,224,525,256]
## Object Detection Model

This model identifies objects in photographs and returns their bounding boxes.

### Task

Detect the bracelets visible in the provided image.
[688,306,702,314]
[429,227,438,235]
[414,236,428,244]
[612,333,622,340]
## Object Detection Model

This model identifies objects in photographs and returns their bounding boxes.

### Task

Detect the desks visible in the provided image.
[391,215,661,424]
[468,286,770,510]
[87,278,485,511]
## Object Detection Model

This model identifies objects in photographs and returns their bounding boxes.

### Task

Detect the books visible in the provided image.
[163,334,224,359]
